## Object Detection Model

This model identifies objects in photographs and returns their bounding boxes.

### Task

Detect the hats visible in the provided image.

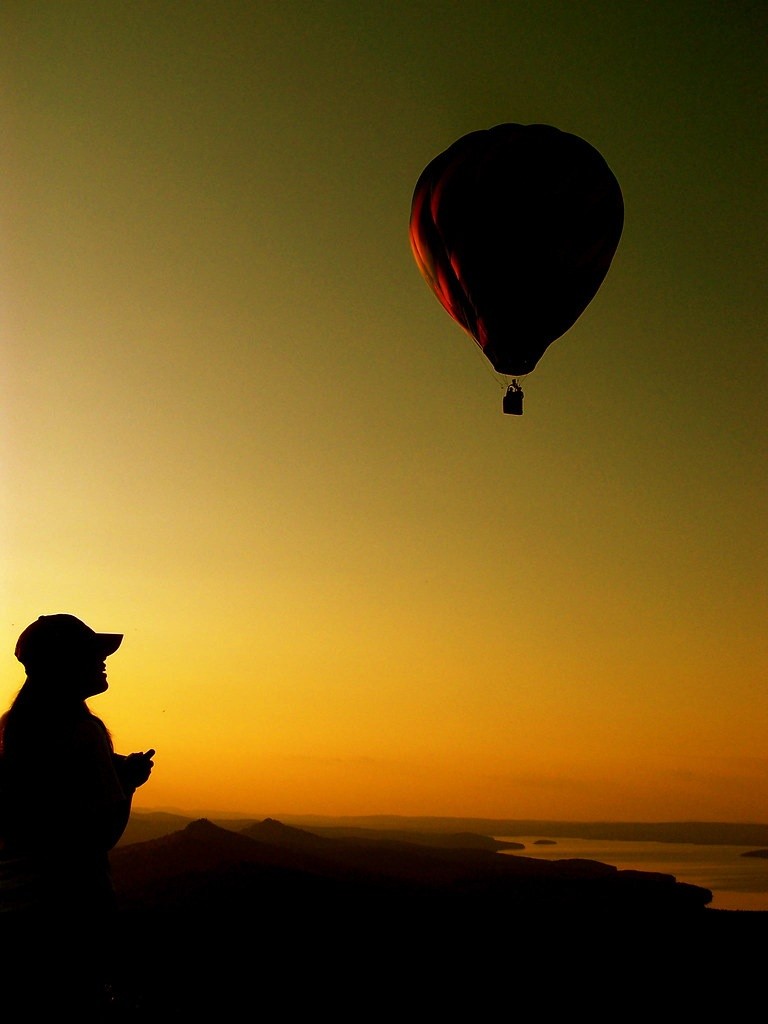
[14,614,124,664]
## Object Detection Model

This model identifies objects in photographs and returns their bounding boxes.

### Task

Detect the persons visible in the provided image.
[0,614,157,1024]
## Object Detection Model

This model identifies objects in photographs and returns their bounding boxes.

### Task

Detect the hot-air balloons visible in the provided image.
[409,123,625,416]
